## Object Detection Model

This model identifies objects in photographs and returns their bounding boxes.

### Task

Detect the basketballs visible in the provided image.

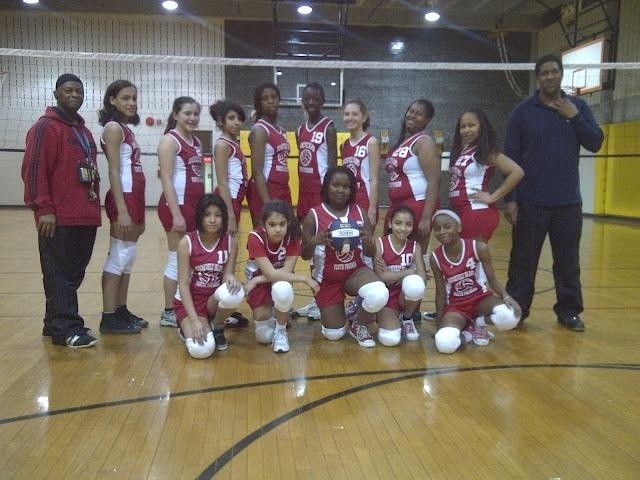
[328,216,360,252]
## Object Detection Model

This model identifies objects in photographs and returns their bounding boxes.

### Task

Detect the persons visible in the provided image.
[171,188,245,358]
[19,71,103,350]
[449,110,525,245]
[155,94,205,328]
[502,54,606,333]
[428,207,522,354]
[295,82,338,224]
[95,77,150,337]
[372,204,429,347]
[298,164,391,349]
[341,100,381,232]
[242,198,320,353]
[386,98,440,254]
[244,83,290,230]
[208,100,248,239]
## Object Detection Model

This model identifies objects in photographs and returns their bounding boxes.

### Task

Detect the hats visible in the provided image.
[55,74,83,89]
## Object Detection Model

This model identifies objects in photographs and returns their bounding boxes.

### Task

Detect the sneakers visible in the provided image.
[294,301,324,320]
[42,325,98,347]
[398,311,421,341]
[272,329,291,353]
[225,312,249,328]
[100,305,149,334]
[557,314,585,331]
[208,321,228,352]
[423,310,436,322]
[160,309,179,328]
[343,302,375,348]
[463,315,495,345]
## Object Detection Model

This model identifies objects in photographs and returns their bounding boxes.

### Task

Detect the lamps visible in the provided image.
[296,0,314,15]
[421,0,441,21]
